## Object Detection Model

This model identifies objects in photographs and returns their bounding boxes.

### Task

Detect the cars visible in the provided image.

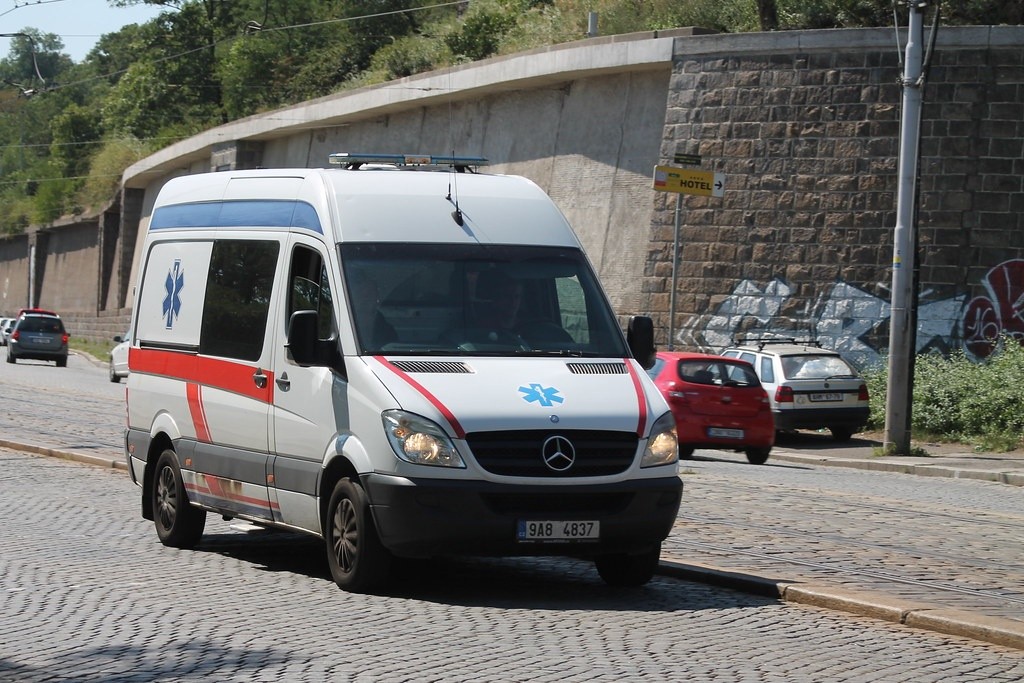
[2,320,17,346]
[7,313,71,367]
[108,329,130,382]
[15,309,59,323]
[704,342,871,443]
[0,318,9,329]
[642,351,774,465]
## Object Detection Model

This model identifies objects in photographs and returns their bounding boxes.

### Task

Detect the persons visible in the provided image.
[352,272,399,349]
[482,278,555,341]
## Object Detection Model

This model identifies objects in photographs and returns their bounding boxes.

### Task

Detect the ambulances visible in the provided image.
[124,152,683,592]
